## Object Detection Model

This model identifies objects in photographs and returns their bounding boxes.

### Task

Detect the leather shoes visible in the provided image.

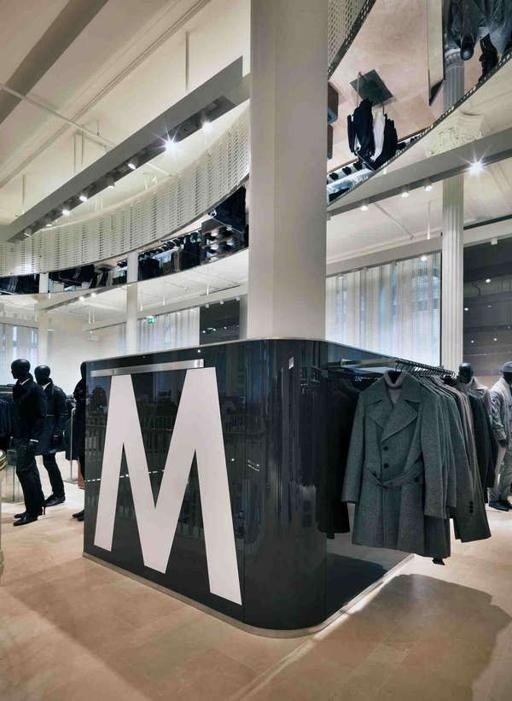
[13,511,39,526]
[73,510,84,522]
[45,493,65,507]
[489,500,512,511]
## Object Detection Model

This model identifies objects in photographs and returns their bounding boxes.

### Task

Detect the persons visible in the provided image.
[9,359,86,526]
[455,361,512,511]
